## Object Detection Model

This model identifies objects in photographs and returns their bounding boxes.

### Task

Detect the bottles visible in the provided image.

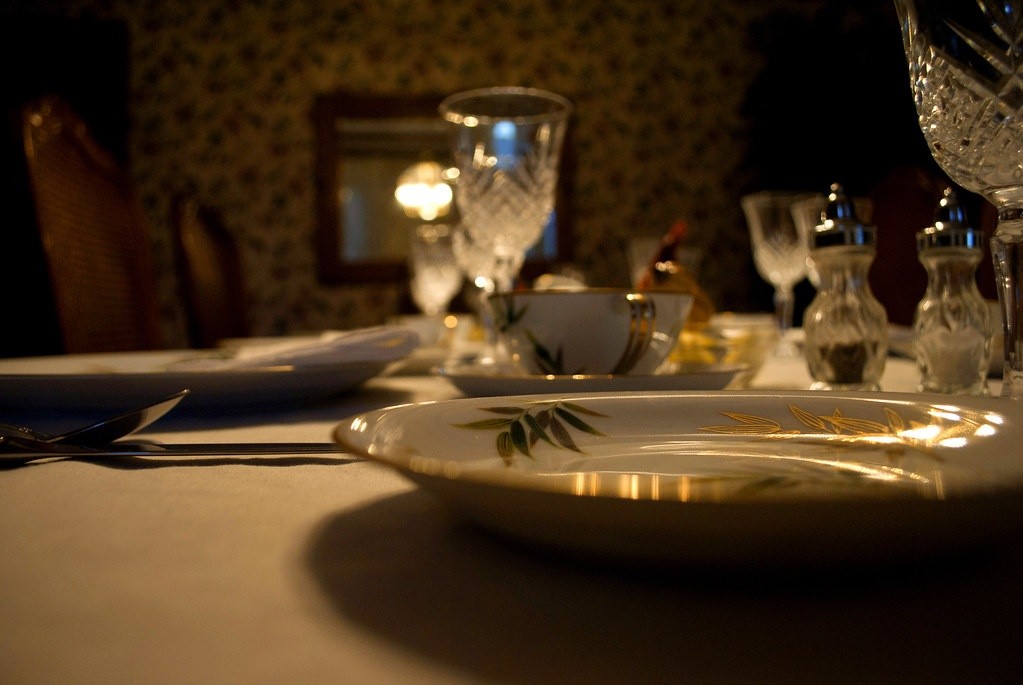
[912,188,993,397]
[802,184,890,392]
[632,219,689,292]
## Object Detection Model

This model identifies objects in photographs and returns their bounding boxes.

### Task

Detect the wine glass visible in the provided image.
[739,191,809,361]
[439,86,571,366]
[893,0,1023,402]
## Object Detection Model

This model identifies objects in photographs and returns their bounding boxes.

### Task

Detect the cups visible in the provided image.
[677,322,780,388]
[482,287,696,375]
[387,316,474,352]
[404,237,464,315]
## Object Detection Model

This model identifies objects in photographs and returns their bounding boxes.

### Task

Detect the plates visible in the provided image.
[428,354,751,399]
[331,390,1023,566]
[1,350,413,413]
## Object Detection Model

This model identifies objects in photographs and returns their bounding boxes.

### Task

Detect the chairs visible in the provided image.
[20,103,163,357]
[176,199,253,350]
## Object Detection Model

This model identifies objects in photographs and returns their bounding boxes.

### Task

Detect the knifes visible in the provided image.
[1,442,356,459]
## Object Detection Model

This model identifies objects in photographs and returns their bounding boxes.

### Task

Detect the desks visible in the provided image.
[1,328,1023,685]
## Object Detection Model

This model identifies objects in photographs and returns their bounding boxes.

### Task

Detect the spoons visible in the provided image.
[0,388,190,451]
